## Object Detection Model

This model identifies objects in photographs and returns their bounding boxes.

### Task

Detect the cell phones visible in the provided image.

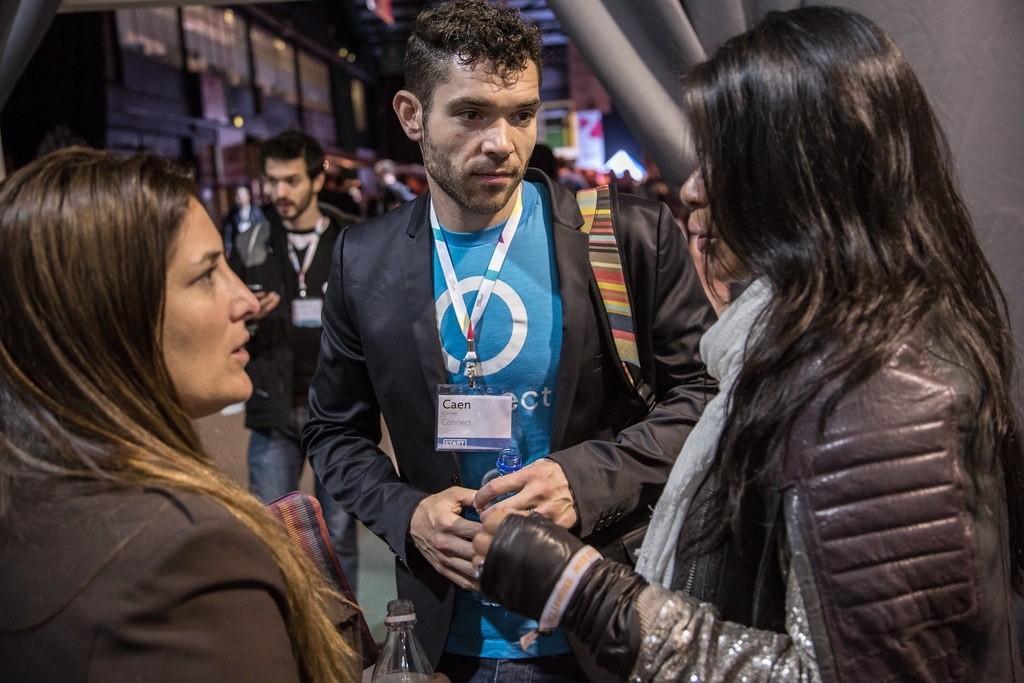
[247,284,265,291]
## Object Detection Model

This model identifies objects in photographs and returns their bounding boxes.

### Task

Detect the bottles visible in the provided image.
[371,599,435,683]
[473,447,525,607]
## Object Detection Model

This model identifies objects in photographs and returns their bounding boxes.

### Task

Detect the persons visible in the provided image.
[220,130,367,607]
[215,145,747,431]
[472,8,1024,683]
[299,0,722,683]
[0,145,451,683]
